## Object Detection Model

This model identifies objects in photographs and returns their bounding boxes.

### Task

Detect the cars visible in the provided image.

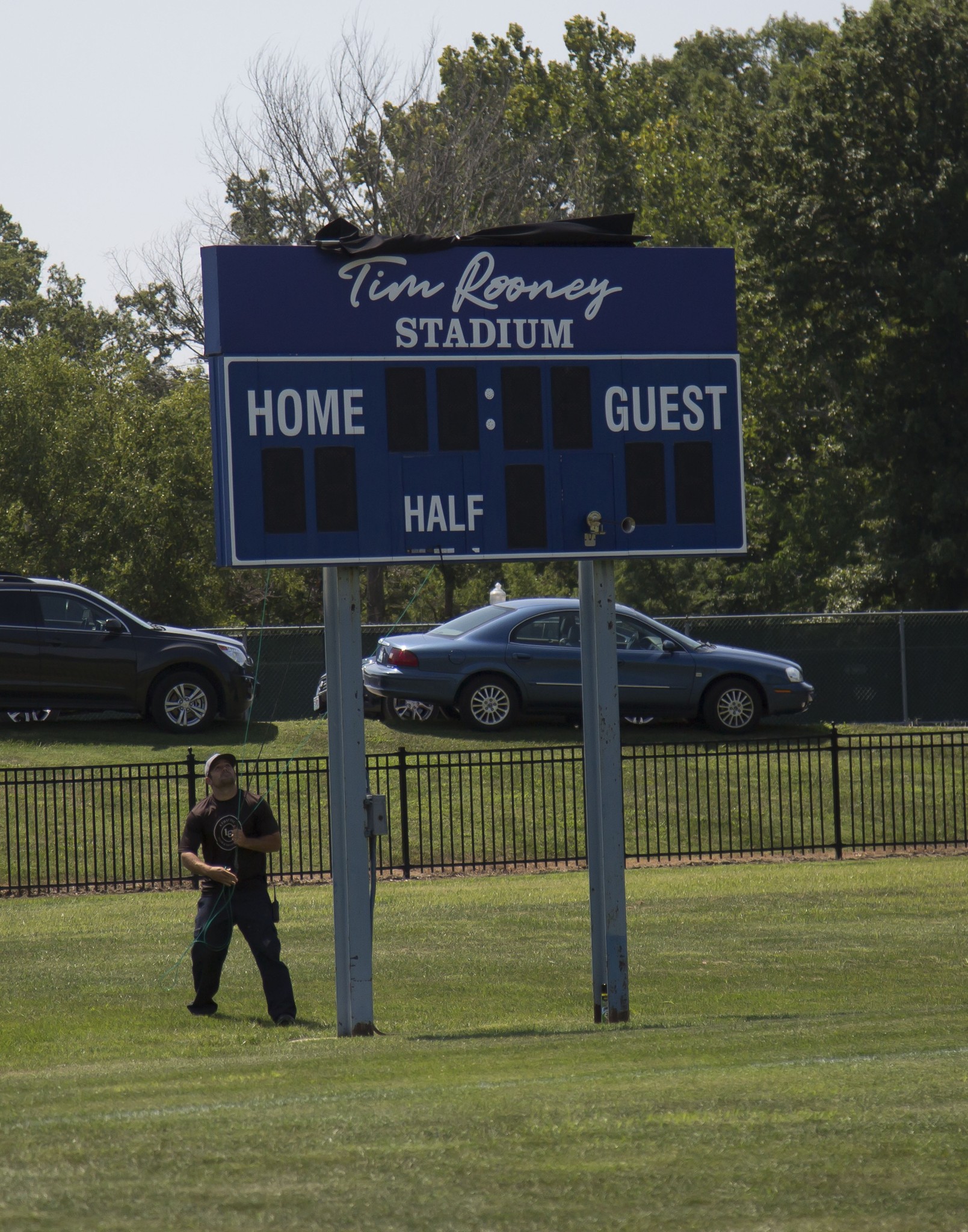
[315,653,659,728]
[364,600,813,735]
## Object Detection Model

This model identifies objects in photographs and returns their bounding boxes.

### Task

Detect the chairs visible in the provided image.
[558,618,581,648]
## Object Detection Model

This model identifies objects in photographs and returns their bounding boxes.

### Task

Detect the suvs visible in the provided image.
[0,575,255,732]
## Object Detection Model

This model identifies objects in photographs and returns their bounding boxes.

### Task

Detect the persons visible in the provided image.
[178,753,297,1027]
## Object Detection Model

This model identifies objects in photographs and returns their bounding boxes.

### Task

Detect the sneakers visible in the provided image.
[275,1014,295,1027]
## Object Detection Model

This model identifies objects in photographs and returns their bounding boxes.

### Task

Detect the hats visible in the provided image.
[205,753,236,778]
